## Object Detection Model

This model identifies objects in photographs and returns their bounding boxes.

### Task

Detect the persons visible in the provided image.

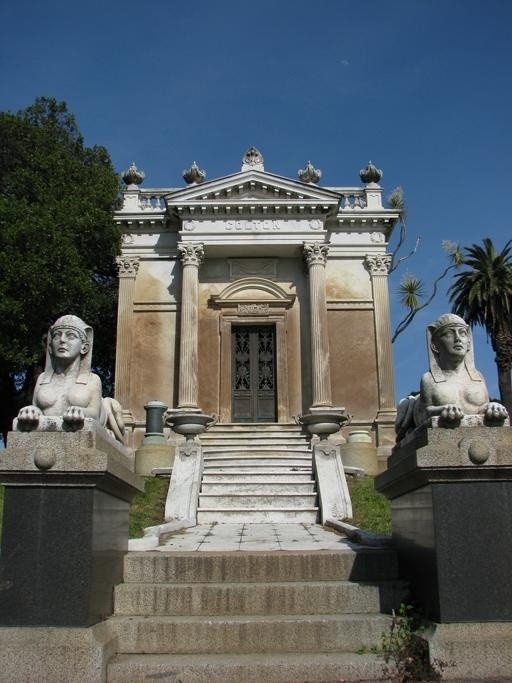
[416,312,508,423]
[17,310,106,428]
